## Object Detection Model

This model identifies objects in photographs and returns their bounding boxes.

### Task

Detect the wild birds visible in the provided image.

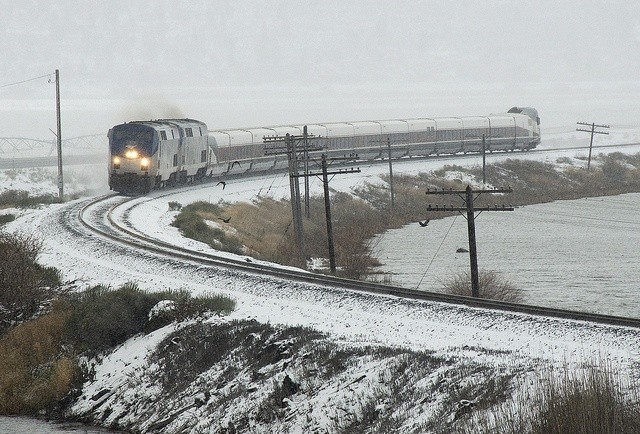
[231,161,242,169]
[419,218,430,226]
[216,181,226,190]
[218,217,233,222]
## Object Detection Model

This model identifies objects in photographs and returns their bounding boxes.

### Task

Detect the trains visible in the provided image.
[107,107,541,194]
